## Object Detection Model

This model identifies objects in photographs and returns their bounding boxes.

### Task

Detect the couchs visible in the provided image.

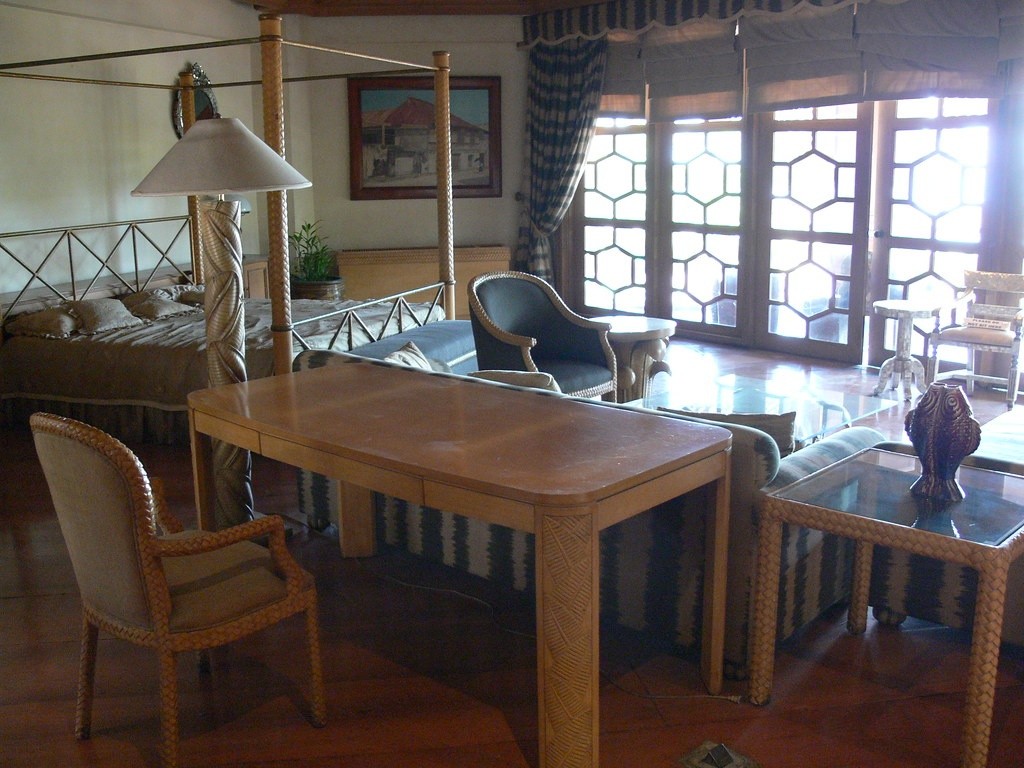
[868,406,1024,646]
[293,350,885,680]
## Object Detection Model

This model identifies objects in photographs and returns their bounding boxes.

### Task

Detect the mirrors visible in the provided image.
[172,62,222,140]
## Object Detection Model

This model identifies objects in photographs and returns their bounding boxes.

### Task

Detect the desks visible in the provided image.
[867,298,936,402]
[186,362,734,768]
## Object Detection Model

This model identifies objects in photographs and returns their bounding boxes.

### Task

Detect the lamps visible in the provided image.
[224,193,251,259]
[130,113,314,548]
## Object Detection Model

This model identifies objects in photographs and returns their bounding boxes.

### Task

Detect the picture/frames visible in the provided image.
[347,75,503,201]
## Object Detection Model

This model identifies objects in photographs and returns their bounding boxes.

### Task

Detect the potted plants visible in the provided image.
[288,219,345,300]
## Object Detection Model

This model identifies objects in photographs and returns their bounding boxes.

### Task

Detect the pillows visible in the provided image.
[131,294,204,321]
[61,298,144,335]
[177,291,204,307]
[123,284,204,309]
[467,370,563,393]
[383,341,432,370]
[4,307,77,339]
[656,406,797,458]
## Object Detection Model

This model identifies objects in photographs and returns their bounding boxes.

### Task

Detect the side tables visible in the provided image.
[589,315,677,404]
[747,447,1024,768]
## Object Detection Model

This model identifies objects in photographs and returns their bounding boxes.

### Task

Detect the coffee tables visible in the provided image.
[669,372,899,443]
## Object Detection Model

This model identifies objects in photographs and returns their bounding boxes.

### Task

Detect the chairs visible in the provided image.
[466,270,618,404]
[925,269,1024,412]
[29,411,328,768]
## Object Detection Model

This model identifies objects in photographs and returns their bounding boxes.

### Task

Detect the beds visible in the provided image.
[0,12,457,465]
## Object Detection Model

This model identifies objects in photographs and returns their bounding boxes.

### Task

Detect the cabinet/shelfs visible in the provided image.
[0,252,271,325]
